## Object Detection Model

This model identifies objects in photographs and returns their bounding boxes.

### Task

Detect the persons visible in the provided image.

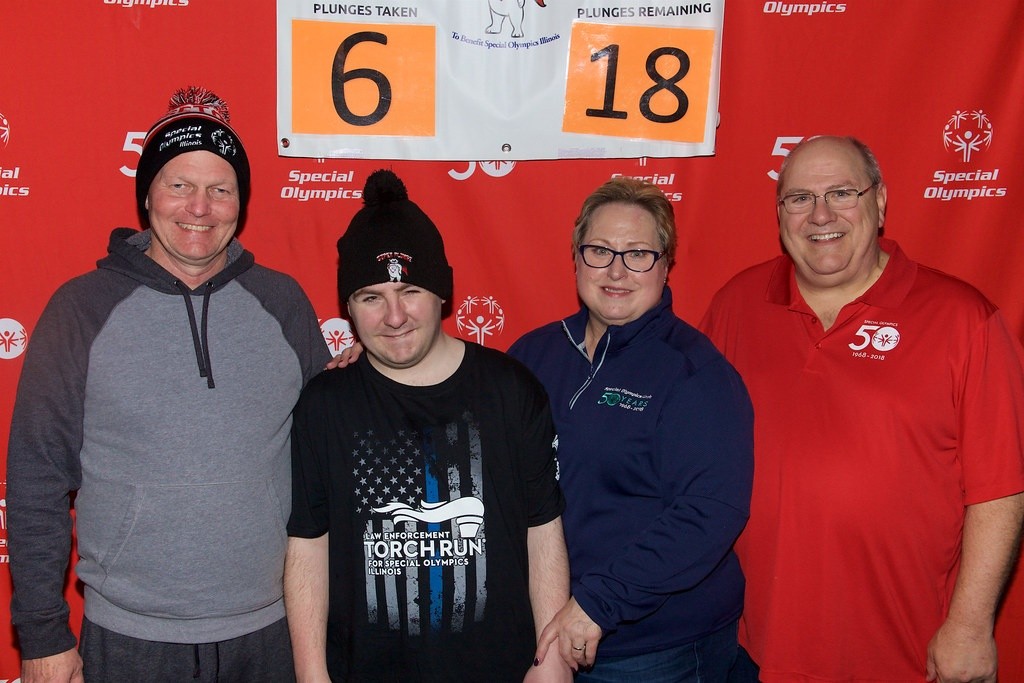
[6,87,336,682]
[282,168,573,683]
[696,133,1023,683]
[498,173,761,682]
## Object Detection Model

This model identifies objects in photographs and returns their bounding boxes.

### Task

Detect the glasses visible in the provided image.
[576,243,670,272]
[777,182,880,214]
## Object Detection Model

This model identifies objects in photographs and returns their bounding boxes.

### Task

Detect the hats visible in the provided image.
[336,173,454,304]
[137,85,249,225]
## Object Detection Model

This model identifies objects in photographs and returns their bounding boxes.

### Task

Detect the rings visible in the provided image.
[571,642,587,652]
